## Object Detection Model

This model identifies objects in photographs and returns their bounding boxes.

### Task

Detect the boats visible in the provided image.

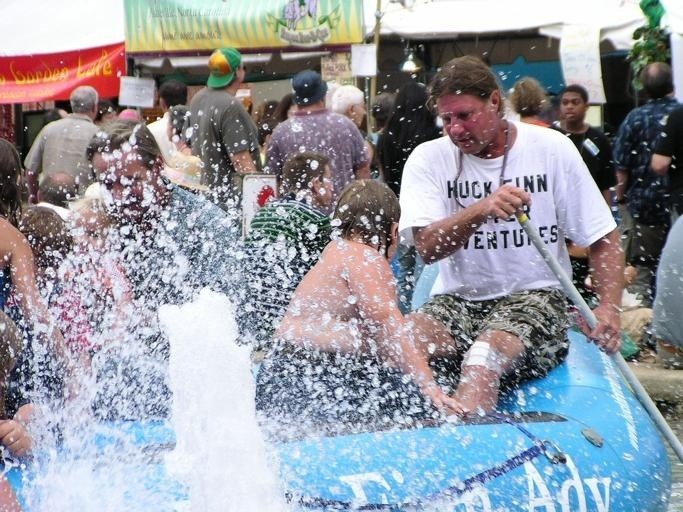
[0,327,671,512]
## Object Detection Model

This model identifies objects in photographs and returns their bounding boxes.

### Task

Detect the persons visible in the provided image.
[254,180,469,421]
[189,49,372,216]
[399,57,626,414]
[26,81,295,420]
[511,77,618,287]
[371,77,442,195]
[251,151,336,334]
[614,62,682,371]
[1,137,88,512]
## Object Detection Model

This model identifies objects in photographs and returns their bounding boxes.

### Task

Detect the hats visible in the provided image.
[292,69,327,107]
[205,47,242,90]
